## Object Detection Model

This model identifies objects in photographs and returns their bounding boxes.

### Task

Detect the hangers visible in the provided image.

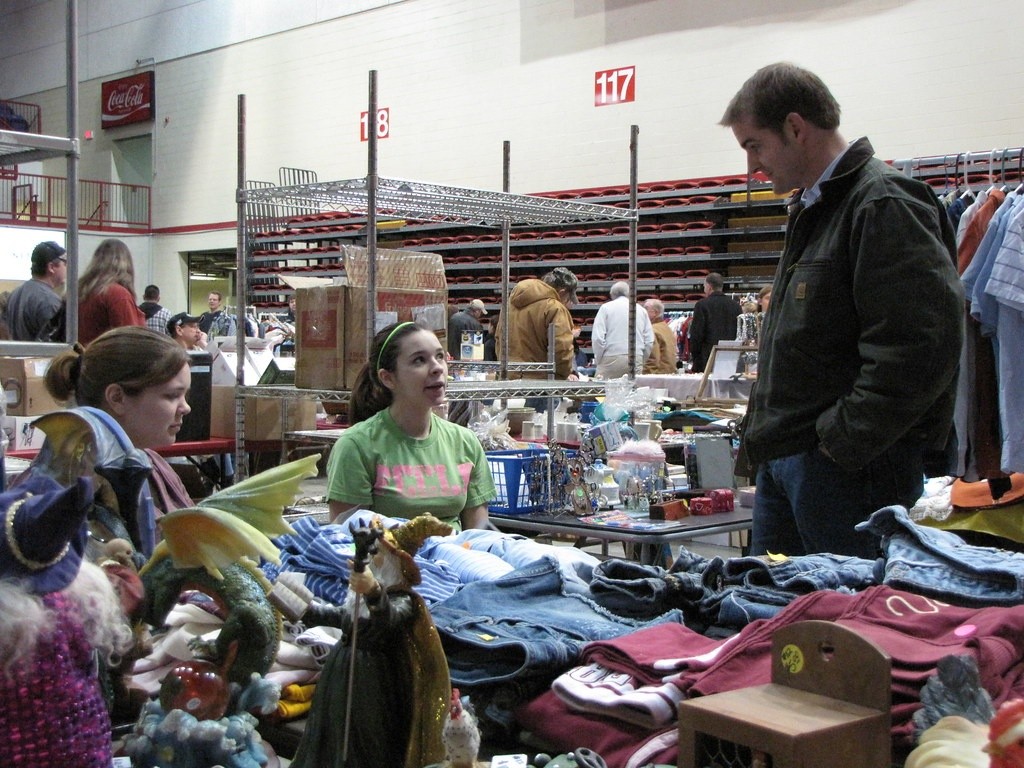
[941,154,950,196]
[957,151,976,202]
[998,147,1011,193]
[984,148,1000,195]
[952,151,966,196]
[916,157,925,181]
[1012,148,1024,197]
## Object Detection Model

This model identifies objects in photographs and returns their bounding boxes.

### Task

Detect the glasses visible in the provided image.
[56,256,68,266]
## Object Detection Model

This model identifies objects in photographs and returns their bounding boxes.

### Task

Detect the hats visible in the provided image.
[553,266,579,305]
[167,311,205,334]
[30,241,66,264]
[0,465,93,596]
[470,299,487,315]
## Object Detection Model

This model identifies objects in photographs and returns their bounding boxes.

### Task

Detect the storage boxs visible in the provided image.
[482,442,581,512]
[207,335,275,387]
[589,423,622,455]
[255,356,296,385]
[0,407,46,451]
[0,352,67,416]
[211,383,317,441]
[278,244,448,391]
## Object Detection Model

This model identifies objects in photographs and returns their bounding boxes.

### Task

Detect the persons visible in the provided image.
[287,517,452,768]
[324,322,497,529]
[0,471,152,768]
[716,64,965,559]
[447,267,777,380]
[0,237,225,355]
[17,325,194,548]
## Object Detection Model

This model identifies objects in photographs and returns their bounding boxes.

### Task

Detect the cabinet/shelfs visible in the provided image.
[251,175,789,374]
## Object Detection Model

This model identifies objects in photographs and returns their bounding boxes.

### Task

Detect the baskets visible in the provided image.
[483,446,583,515]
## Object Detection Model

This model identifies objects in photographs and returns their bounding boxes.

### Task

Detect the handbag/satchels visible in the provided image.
[34,301,66,343]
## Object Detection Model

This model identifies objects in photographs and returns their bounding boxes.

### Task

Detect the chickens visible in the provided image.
[440,687,480,768]
[904,653,1024,767]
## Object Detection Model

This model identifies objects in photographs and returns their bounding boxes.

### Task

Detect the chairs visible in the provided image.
[674,619,894,768]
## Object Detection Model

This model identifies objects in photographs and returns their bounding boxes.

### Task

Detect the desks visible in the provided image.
[488,506,754,567]
